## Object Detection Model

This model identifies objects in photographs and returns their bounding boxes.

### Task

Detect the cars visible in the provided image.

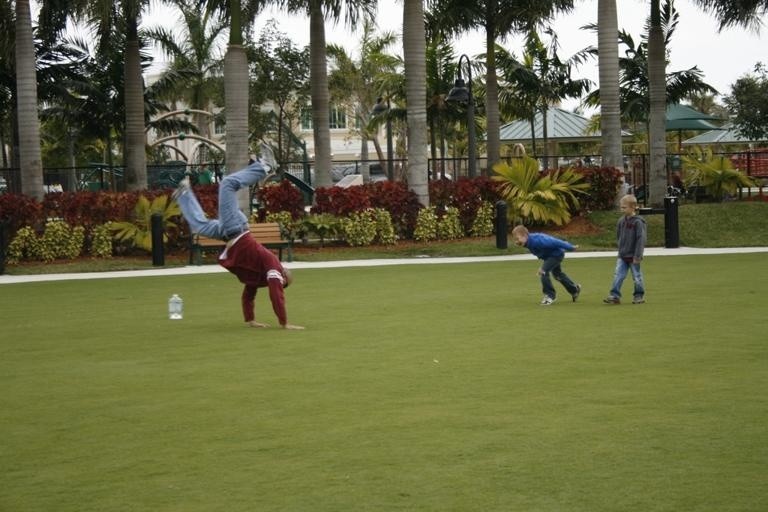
[728,148,767,176]
[288,163,451,192]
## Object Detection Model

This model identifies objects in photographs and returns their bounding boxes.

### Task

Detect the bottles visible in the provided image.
[168,293,185,321]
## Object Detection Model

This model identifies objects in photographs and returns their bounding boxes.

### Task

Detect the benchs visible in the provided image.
[188,220,293,266]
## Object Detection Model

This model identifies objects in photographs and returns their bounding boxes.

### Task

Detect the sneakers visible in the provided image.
[170,179,189,200]
[540,296,556,305]
[602,295,620,304]
[259,142,275,174]
[572,284,580,302]
[631,296,643,303]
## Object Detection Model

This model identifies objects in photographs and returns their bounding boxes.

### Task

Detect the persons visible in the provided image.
[175,141,306,331]
[510,221,582,307]
[602,193,644,307]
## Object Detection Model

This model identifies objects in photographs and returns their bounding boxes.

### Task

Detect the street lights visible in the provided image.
[444,54,481,183]
